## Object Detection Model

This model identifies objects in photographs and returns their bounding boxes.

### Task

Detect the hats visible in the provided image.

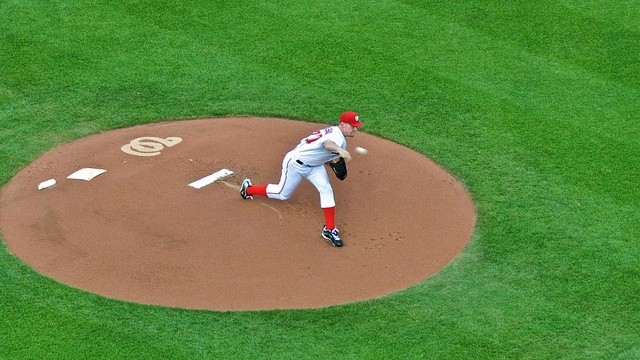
[340,112,363,127]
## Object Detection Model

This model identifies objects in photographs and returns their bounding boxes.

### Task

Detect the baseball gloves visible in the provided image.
[329,157,347,181]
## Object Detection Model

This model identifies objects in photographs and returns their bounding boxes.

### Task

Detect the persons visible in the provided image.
[240,112,364,247]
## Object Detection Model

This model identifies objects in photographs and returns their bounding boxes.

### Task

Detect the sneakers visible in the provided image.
[240,179,253,200]
[322,225,342,247]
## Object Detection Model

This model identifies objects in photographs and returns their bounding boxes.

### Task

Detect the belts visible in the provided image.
[296,159,313,167]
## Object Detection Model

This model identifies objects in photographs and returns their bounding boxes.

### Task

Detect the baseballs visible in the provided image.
[355,146,367,155]
